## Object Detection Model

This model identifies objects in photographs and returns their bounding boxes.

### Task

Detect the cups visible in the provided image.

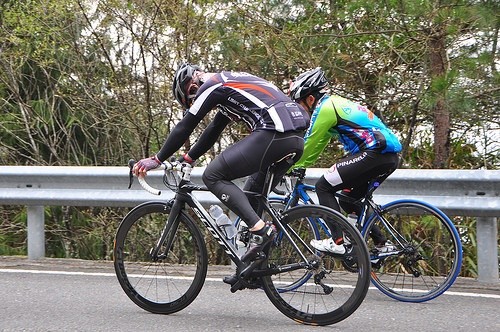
[236,221,249,246]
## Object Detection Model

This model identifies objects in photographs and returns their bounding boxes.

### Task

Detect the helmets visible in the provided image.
[173,62,204,109]
[289,66,329,101]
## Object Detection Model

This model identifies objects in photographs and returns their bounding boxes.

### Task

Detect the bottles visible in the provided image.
[209,205,238,239]
[343,212,358,244]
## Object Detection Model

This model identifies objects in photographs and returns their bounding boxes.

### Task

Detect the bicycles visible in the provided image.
[230,165,463,303]
[112,151,371,326]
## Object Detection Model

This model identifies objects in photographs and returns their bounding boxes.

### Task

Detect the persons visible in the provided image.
[132,62,310,290]
[282,66,402,256]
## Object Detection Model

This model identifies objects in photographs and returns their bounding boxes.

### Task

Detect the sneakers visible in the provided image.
[310,238,346,254]
[241,223,277,263]
[223,269,264,290]
[369,240,397,263]
